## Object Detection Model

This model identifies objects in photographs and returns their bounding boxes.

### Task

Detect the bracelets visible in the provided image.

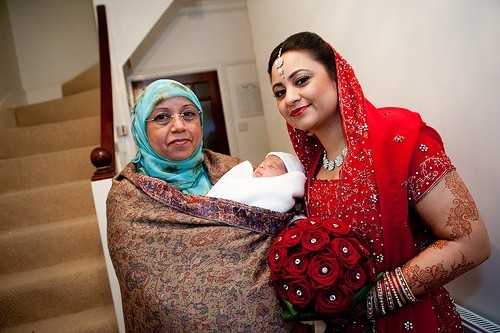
[367,266,420,319]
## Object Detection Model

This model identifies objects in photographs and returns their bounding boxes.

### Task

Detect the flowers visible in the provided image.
[268,218,384,318]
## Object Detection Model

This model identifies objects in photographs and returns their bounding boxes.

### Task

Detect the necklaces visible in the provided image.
[322,126,355,171]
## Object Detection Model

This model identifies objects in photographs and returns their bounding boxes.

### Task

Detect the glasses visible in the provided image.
[145,107,201,122]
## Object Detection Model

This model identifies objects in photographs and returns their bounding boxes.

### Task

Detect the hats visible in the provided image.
[265,151,306,177]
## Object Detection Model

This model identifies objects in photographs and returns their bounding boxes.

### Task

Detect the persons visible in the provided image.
[267,32,491,332]
[206,149,307,229]
[106,78,292,332]
